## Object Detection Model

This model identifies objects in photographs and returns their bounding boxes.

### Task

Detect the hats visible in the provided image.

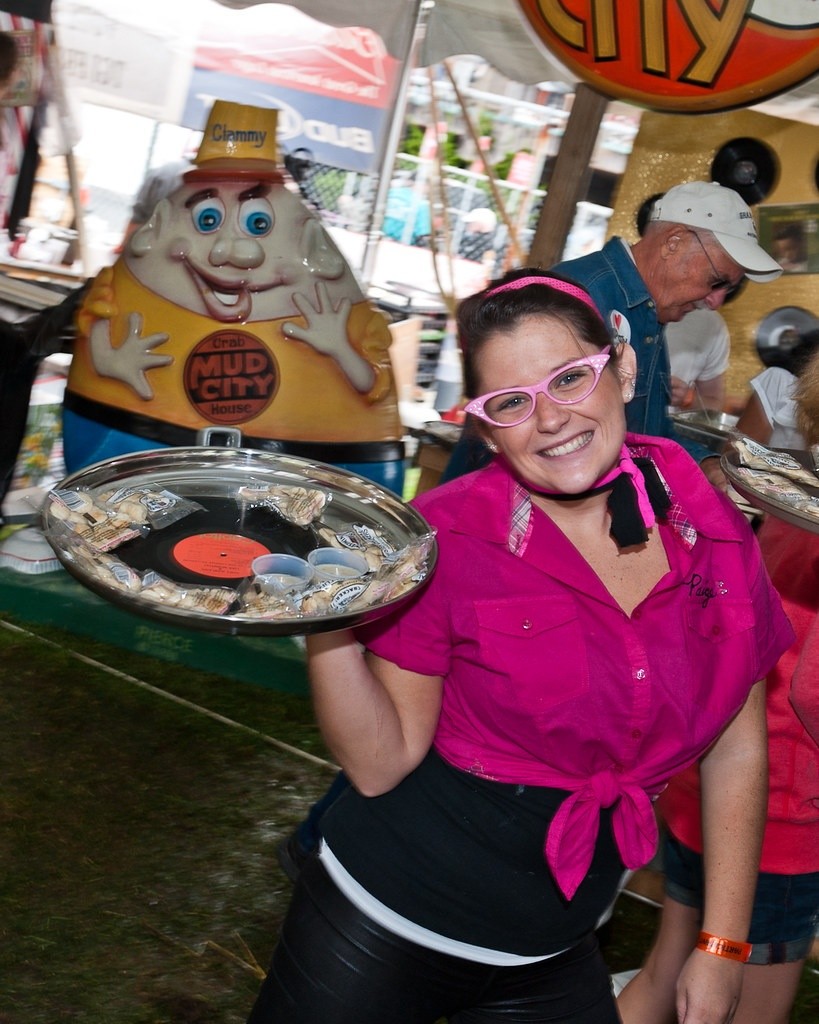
[649,181,784,283]
[462,208,497,228]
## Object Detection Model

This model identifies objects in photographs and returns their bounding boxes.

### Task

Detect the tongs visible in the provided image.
[675,381,712,426]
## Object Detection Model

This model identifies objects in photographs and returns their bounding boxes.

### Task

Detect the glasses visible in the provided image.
[687,229,739,294]
[464,344,612,427]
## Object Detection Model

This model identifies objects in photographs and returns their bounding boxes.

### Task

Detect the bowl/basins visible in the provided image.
[307,546,369,587]
[251,552,315,590]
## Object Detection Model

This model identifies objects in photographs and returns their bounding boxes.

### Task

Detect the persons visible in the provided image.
[441,179,785,517]
[735,336,819,473]
[633,192,732,423]
[249,268,787,1024]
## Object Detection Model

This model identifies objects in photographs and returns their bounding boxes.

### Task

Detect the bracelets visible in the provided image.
[696,931,753,963]
